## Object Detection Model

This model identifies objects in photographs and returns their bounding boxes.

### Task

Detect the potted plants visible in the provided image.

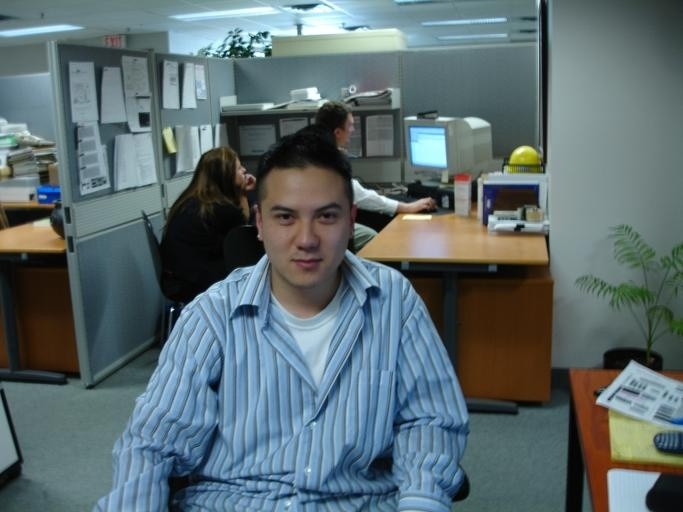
[573,222,683,370]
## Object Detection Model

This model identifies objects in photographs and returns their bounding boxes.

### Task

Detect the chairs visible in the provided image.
[140,209,194,347]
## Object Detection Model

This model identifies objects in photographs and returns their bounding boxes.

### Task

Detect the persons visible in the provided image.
[92,127,471,511]
[314,100,439,257]
[158,145,265,304]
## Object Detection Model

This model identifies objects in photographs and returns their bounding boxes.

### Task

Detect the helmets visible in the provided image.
[507,145,541,173]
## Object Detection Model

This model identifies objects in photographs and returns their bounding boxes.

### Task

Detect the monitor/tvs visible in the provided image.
[403,116,492,188]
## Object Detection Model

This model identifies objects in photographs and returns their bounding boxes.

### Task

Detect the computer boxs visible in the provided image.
[407,180,477,209]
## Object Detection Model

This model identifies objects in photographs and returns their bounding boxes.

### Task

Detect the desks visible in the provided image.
[353,202,553,414]
[566,366,683,512]
[0,199,79,385]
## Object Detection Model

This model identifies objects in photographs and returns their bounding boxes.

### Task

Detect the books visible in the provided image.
[221,102,274,111]
[287,86,394,109]
[269,100,296,109]
[0,117,58,180]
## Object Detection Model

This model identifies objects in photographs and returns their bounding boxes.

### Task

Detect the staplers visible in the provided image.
[417,111,438,119]
